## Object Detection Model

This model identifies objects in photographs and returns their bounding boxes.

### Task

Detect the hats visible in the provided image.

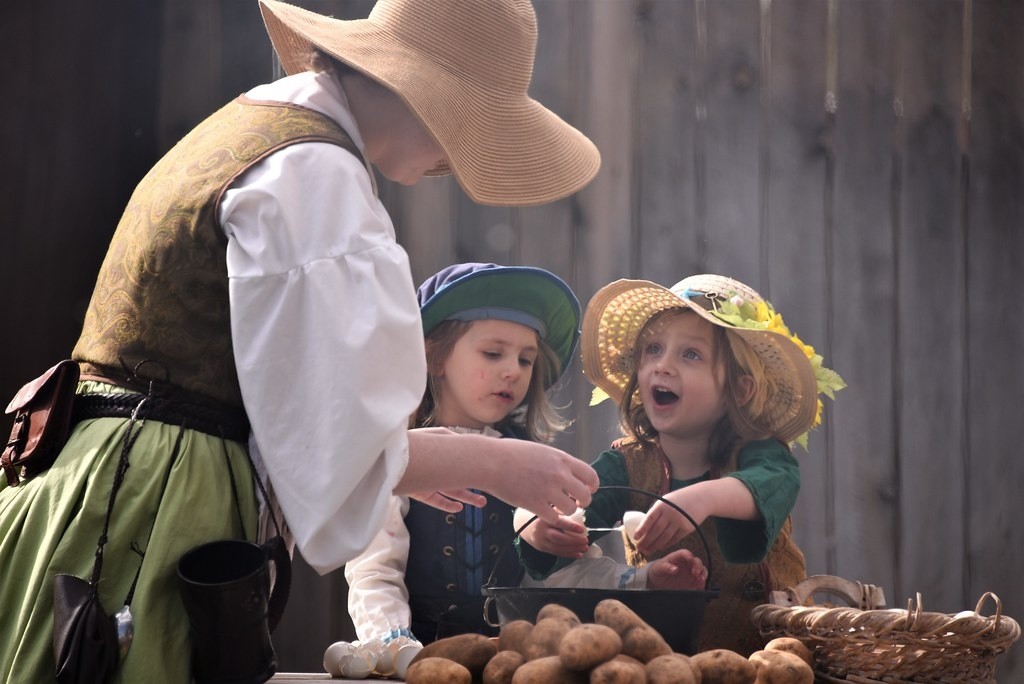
[415,262,580,408]
[581,273,818,444]
[258,0,603,207]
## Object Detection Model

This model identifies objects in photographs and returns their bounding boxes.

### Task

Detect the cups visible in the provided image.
[177,537,292,684]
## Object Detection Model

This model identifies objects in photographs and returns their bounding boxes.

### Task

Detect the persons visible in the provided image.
[513,275,847,660]
[405,262,583,655]
[0,0,599,683]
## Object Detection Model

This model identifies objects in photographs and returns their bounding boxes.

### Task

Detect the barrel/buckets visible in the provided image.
[483,485,715,657]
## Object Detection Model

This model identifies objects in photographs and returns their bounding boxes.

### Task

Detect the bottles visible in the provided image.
[115,604,134,668]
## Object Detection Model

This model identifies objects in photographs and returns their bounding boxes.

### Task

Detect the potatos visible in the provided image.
[406,599,816,684]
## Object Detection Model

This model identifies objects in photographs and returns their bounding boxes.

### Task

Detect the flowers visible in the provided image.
[587,282,848,453]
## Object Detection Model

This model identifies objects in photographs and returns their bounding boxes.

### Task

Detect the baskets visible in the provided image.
[751,573,1021,684]
